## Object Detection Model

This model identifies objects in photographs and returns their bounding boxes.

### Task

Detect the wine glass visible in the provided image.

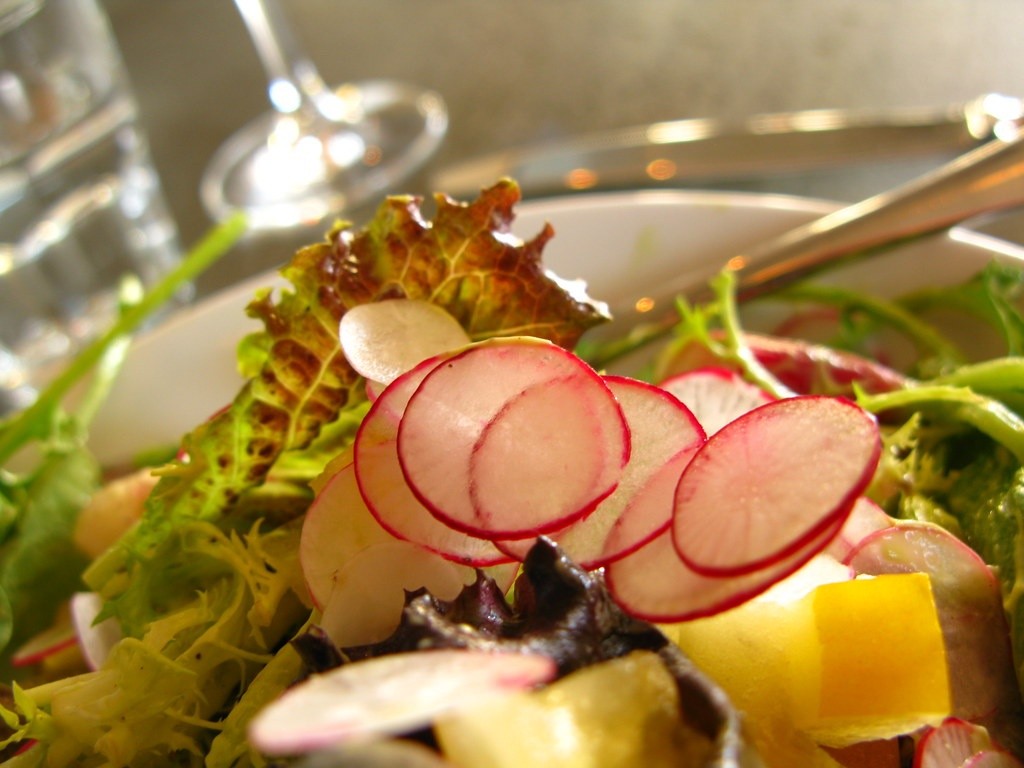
[201,0,447,230]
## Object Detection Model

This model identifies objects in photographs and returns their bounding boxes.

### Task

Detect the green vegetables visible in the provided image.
[0,177,1024,768]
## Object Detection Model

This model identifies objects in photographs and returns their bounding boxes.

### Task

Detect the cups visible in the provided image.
[0,0,193,402]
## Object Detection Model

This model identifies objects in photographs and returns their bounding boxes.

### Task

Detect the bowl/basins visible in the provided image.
[0,193,1023,767]
